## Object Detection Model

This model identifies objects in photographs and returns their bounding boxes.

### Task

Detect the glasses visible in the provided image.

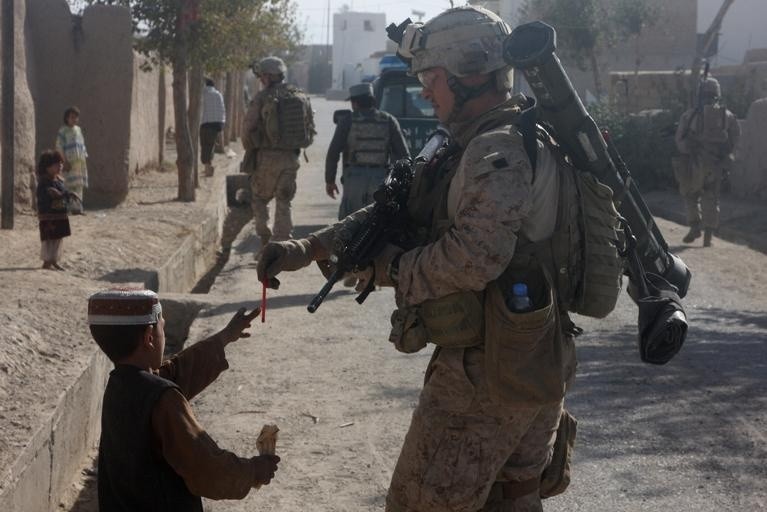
[418,71,438,89]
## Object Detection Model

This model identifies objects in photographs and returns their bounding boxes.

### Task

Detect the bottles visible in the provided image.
[507,284,535,314]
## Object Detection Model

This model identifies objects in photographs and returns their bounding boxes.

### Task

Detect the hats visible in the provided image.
[87,287,163,325]
[344,83,373,101]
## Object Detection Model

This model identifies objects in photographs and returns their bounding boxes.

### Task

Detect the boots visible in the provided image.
[684,224,712,247]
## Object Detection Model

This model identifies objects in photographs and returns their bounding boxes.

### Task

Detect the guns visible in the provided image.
[308,155,419,313]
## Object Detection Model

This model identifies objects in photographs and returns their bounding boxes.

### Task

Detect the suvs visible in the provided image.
[333,66,439,167]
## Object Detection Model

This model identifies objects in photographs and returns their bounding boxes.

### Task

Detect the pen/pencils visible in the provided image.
[262,274,268,323]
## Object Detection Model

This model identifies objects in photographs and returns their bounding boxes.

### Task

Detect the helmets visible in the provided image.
[406,4,514,93]
[696,77,720,97]
[253,56,287,76]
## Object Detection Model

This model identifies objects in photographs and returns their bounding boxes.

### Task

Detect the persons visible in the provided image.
[87,284,281,511]
[674,77,741,248]
[34,147,88,272]
[239,54,315,249]
[255,4,625,512]
[324,81,411,295]
[55,104,90,216]
[198,75,227,179]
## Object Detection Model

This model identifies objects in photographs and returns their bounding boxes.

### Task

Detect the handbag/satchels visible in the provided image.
[485,251,566,405]
